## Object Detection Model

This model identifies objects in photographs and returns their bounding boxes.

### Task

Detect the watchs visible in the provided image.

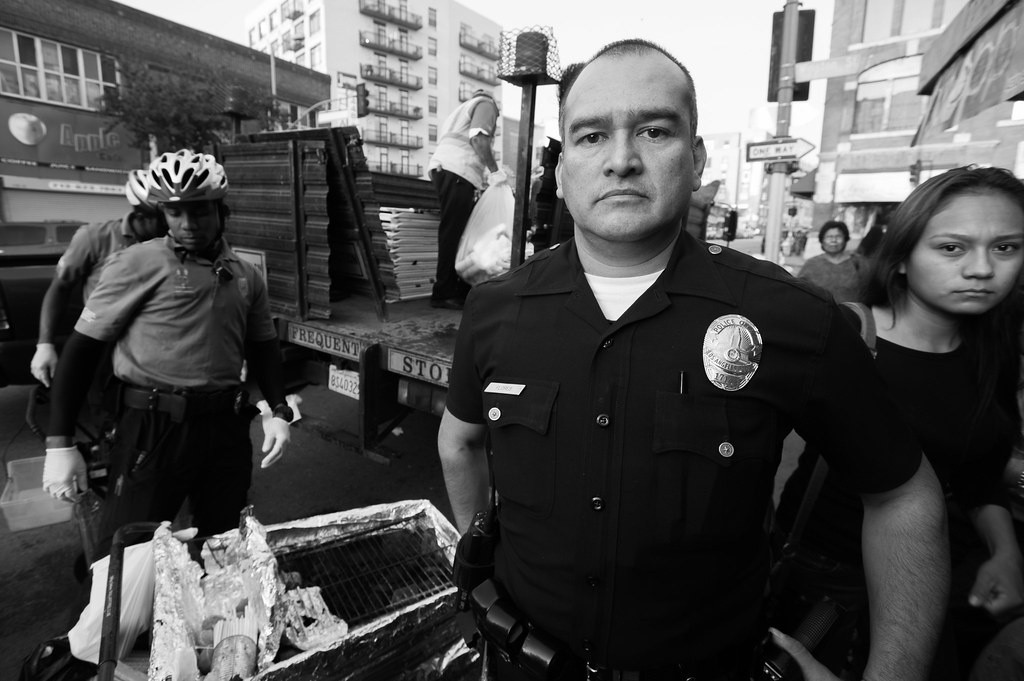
[274,407,293,421]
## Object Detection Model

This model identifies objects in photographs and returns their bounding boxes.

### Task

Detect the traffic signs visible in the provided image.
[744,137,817,163]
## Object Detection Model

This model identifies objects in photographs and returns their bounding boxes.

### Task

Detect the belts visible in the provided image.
[453,541,647,680]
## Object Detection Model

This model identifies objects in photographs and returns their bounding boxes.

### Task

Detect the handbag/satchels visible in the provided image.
[757,539,865,681]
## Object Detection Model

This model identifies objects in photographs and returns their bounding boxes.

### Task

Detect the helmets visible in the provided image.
[148,150,229,201]
[472,89,500,112]
[126,168,153,210]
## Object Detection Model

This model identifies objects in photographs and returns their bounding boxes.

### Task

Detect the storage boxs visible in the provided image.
[0,456,77,532]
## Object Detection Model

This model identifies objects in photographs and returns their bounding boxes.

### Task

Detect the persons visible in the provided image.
[736,371,824,375]
[437,42,951,681]
[777,168,1024,681]
[429,89,499,308]
[797,221,871,305]
[30,148,293,656]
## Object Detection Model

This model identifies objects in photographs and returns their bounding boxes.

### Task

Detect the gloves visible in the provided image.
[262,416,288,468]
[119,382,256,419]
[43,446,88,502]
[31,344,59,388]
